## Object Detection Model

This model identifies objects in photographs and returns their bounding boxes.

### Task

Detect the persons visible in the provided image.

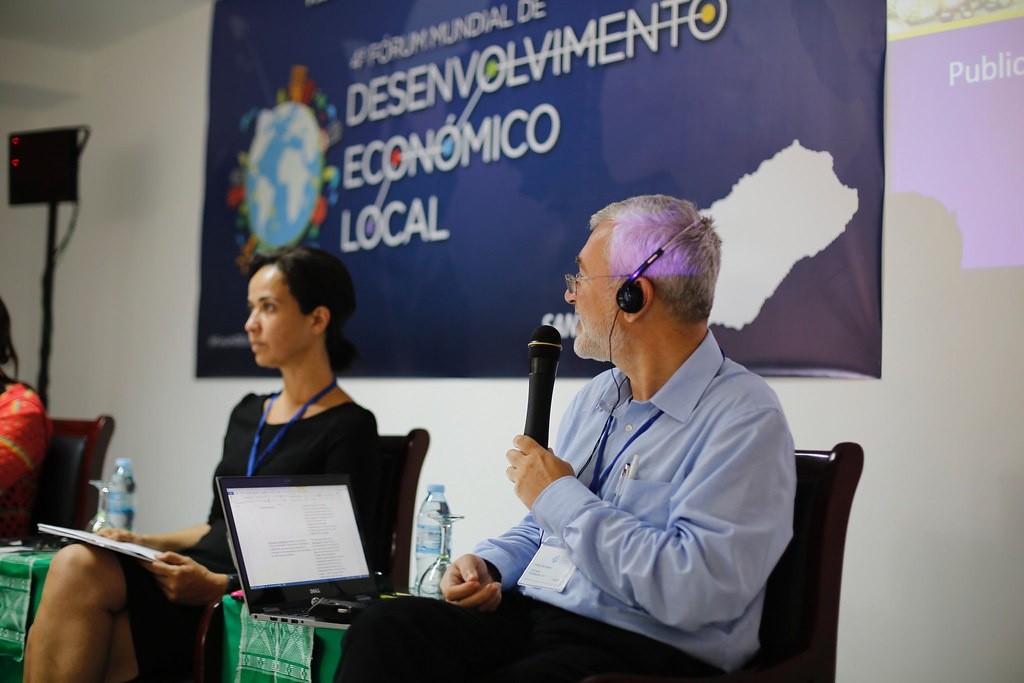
[332,195,797,683]
[24,248,390,683]
[0,299,52,537]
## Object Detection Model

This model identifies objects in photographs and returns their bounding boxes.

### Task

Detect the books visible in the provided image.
[36,522,165,563]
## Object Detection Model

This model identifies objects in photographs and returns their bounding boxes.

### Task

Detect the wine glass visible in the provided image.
[428,513,464,600]
[86,479,109,533]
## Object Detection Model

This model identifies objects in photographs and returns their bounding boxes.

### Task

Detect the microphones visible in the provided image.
[525,325,563,452]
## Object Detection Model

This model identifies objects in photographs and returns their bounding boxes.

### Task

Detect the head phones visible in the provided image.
[616,219,705,313]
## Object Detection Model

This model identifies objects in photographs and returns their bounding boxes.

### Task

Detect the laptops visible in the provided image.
[216,474,381,631]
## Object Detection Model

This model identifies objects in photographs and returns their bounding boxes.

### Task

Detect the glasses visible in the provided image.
[563,271,631,294]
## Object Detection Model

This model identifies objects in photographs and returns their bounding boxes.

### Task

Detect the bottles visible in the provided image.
[105,456,136,533]
[415,484,451,596]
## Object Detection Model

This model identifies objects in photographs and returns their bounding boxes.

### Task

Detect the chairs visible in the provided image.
[0,417,114,549]
[193,429,430,683]
[583,443,863,683]
